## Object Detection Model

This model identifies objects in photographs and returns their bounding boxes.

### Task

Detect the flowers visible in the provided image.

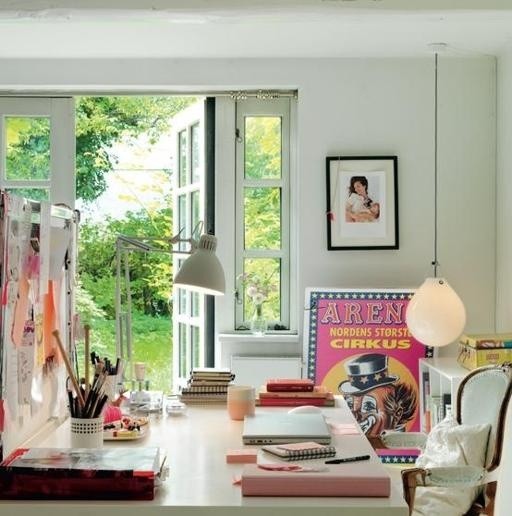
[235,266,279,319]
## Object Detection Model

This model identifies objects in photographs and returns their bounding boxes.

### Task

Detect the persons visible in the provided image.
[337,353,418,438]
[350,202,380,222]
[344,176,377,222]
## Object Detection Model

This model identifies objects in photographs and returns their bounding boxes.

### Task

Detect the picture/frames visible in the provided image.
[325,154,399,250]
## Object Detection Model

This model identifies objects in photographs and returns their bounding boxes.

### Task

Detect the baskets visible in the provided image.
[69,417,105,449]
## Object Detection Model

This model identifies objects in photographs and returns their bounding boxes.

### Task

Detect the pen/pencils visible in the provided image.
[325,454,373,465]
[66,364,111,419]
[89,351,122,376]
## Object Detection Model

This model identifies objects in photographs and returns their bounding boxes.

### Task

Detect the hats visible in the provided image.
[338,353,399,396]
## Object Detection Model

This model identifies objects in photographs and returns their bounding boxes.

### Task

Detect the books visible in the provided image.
[261,442,336,461]
[0,445,162,501]
[182,367,235,402]
[256,378,335,407]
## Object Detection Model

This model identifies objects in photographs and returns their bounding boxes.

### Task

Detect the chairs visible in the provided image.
[375,367,512,516]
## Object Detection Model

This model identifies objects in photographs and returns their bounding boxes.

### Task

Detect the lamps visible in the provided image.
[403,43,467,348]
[114,221,226,390]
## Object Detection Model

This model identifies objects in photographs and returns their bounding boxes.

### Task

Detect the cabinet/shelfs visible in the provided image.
[417,355,471,434]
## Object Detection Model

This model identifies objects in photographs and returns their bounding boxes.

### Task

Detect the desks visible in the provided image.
[2,393,409,516]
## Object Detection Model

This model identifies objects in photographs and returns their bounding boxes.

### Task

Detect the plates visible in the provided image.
[102,418,150,440]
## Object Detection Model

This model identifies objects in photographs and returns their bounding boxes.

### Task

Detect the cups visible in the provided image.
[135,362,146,382]
[226,386,255,421]
